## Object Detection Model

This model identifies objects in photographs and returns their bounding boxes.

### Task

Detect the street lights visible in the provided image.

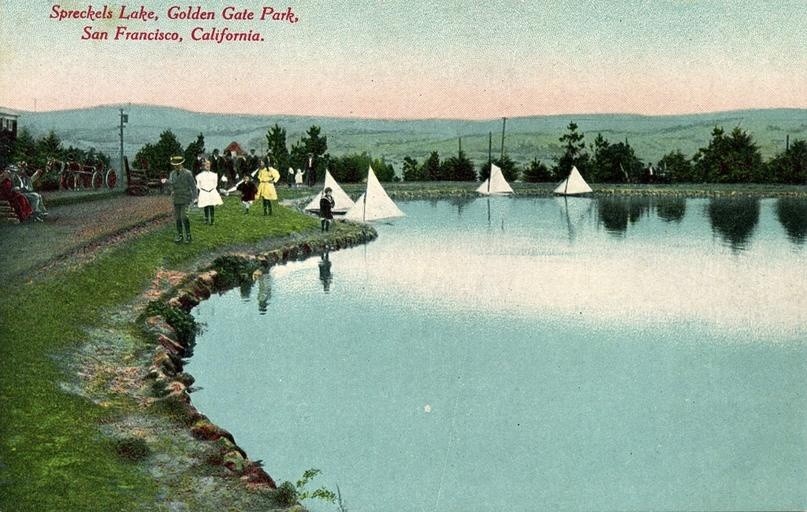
[307,152,313,185]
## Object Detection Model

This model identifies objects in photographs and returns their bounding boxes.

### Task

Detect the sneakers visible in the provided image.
[205,218,215,225]
[264,209,272,215]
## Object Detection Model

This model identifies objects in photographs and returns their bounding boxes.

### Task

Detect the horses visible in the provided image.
[45,156,81,192]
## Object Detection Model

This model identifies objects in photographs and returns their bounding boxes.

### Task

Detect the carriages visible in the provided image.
[44,147,117,190]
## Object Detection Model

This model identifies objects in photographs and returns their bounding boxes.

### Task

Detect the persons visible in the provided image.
[317,185,336,234]
[645,161,657,183]
[285,166,294,188]
[194,156,224,226]
[316,252,332,294]
[13,159,48,224]
[160,154,200,245]
[0,163,32,225]
[254,155,281,215]
[240,284,251,302]
[255,272,272,314]
[293,168,305,188]
[305,153,317,187]
[236,173,259,214]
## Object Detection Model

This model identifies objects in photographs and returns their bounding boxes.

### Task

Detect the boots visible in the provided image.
[175,219,192,244]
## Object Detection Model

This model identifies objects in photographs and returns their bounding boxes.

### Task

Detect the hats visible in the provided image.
[169,154,187,166]
